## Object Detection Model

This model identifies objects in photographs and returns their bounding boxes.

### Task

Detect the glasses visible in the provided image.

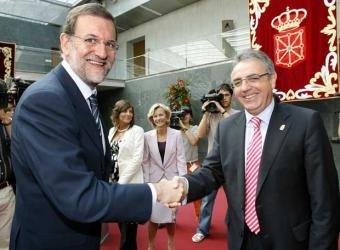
[70,34,119,50]
[231,72,269,89]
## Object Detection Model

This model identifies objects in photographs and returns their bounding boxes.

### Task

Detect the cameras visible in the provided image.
[171,112,184,129]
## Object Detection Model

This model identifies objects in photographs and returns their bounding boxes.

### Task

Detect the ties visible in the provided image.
[244,117,262,235]
[89,94,102,140]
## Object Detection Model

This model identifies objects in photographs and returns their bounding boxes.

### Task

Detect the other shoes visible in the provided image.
[191,233,206,242]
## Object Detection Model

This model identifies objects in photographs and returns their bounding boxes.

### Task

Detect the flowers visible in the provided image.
[161,77,192,111]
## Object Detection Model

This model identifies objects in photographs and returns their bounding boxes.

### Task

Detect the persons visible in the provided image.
[155,50,340,250]
[9,4,185,250]
[0,83,241,250]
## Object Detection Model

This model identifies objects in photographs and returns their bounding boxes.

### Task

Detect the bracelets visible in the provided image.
[221,110,227,115]
[183,128,188,132]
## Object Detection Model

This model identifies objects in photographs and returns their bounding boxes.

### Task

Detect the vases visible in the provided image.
[170,110,192,128]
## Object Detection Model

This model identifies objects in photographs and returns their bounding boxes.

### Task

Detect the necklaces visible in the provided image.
[118,123,130,133]
